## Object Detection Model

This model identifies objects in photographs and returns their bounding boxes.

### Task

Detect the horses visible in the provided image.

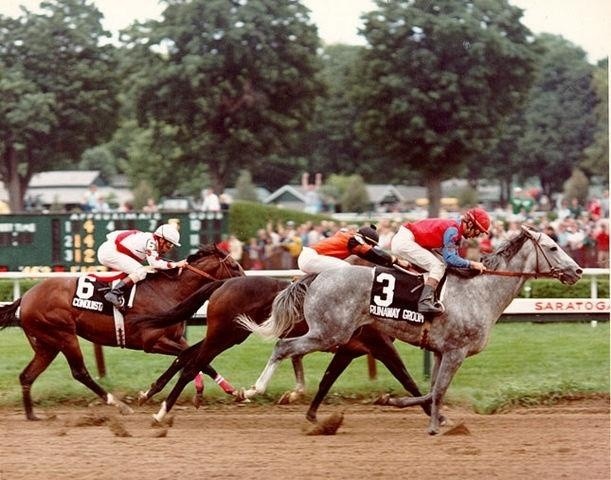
[235,223,582,436]
[0,243,246,421]
[137,256,454,428]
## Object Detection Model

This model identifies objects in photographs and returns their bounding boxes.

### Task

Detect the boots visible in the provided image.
[105,280,128,308]
[417,284,442,313]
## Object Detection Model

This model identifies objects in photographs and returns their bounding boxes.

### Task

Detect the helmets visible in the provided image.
[153,223,181,248]
[358,224,380,246]
[465,208,491,235]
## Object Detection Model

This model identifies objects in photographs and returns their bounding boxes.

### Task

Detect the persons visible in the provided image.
[295,218,414,279]
[195,184,221,212]
[388,206,491,315]
[121,199,135,214]
[85,183,97,207]
[91,192,111,213]
[142,196,158,212]
[94,223,189,316]
[243,218,296,265]
[491,184,606,267]
